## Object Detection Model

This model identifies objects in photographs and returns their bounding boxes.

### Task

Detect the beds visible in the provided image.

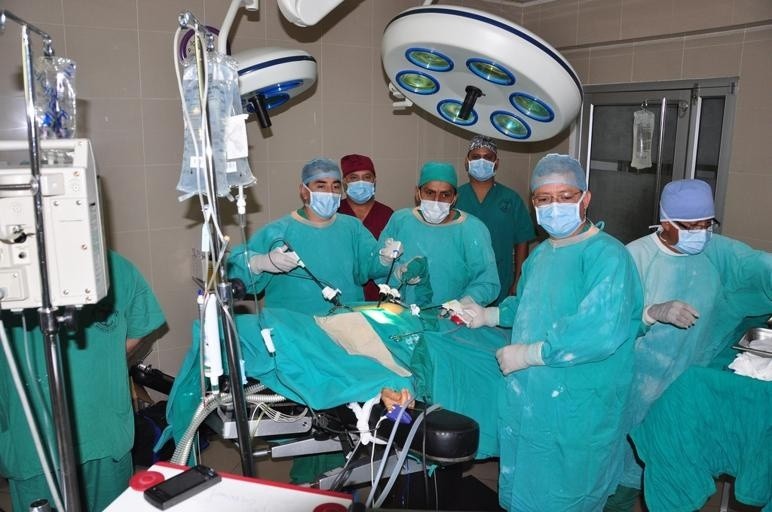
[237,303,512,456]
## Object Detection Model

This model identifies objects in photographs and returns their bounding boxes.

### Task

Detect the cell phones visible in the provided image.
[143,464,221,511]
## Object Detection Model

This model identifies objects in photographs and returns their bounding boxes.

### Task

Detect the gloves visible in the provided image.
[439,295,474,318]
[495,341,546,376]
[396,256,421,285]
[379,238,403,265]
[647,300,699,329]
[249,244,298,274]
[462,303,499,329]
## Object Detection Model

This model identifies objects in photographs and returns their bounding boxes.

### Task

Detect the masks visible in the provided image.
[344,181,375,205]
[466,157,496,182]
[418,189,456,224]
[667,217,713,255]
[531,190,586,239]
[302,182,341,220]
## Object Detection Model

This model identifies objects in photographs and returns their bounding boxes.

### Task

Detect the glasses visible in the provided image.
[532,191,581,206]
[674,218,721,233]
[350,174,373,181]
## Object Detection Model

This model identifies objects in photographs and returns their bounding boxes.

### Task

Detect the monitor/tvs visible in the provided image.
[0,138,111,310]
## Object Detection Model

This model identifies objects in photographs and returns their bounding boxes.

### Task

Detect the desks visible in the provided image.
[687,310,770,510]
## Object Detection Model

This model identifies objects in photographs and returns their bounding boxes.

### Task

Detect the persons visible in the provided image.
[0,246,168,512]
[333,154,394,309]
[368,159,501,323]
[449,134,537,310]
[380,388,416,413]
[452,152,647,512]
[607,171,772,512]
[223,156,403,487]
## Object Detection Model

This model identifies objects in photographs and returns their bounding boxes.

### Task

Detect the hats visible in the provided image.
[658,179,715,223]
[341,155,375,178]
[419,162,457,192]
[466,136,497,156]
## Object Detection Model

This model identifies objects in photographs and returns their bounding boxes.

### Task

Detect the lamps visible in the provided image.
[219,1,586,148]
